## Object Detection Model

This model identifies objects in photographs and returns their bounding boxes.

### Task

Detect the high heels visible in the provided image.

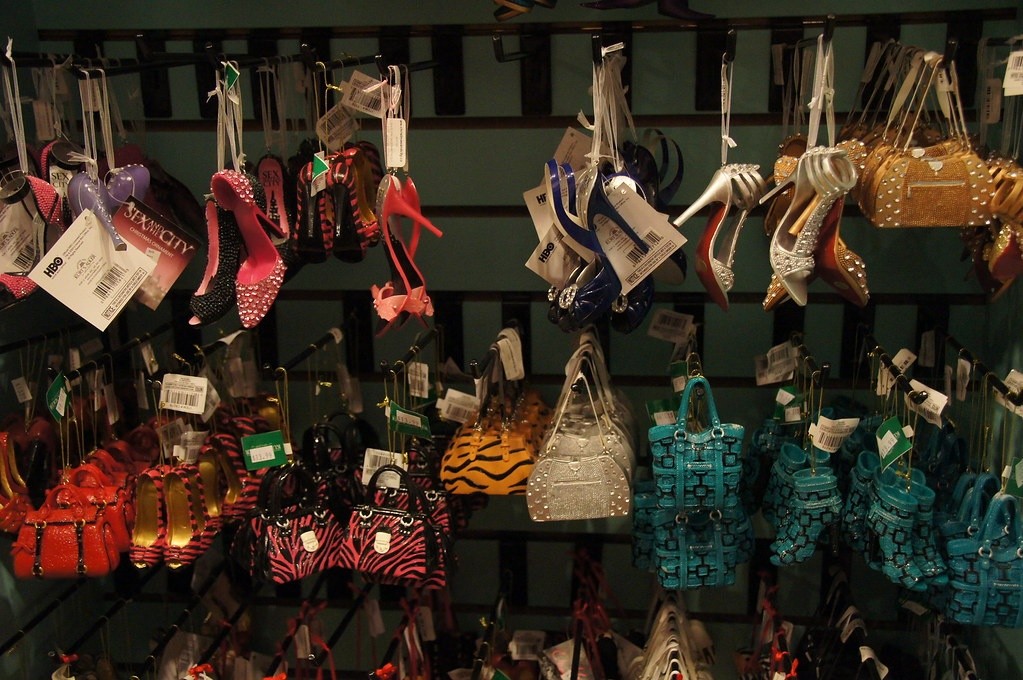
[0,28,869,343]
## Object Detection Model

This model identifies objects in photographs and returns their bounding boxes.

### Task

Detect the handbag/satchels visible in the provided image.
[833,34,991,228]
[0,340,1023,680]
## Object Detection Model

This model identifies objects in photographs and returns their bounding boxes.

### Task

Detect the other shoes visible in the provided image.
[959,151,1023,303]
